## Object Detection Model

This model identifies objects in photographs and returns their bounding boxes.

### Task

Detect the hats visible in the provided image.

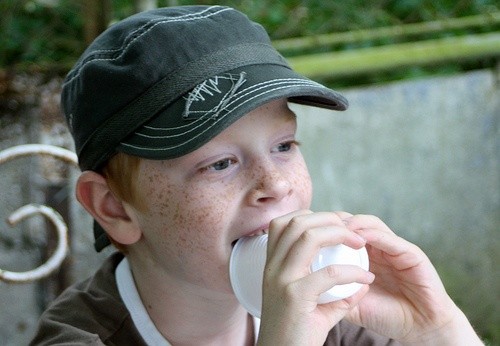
[59,3,348,174]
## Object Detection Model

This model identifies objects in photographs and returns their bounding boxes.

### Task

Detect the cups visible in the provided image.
[229,232,370,319]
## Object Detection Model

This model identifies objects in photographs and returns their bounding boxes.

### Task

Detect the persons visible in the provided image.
[19,90,79,319]
[24,5,486,346]
